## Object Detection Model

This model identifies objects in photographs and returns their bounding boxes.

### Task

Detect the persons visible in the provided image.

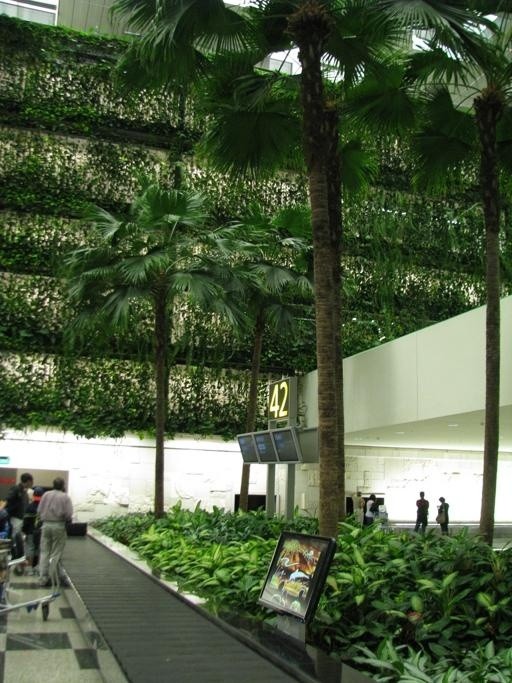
[362,494,379,526]
[1,472,74,588]
[436,497,450,537]
[413,491,429,534]
[351,490,364,527]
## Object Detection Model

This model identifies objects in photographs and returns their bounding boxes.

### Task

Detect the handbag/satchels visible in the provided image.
[436,512,446,523]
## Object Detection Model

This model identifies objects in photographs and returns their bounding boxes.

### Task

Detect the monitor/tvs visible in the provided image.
[235,427,320,465]
